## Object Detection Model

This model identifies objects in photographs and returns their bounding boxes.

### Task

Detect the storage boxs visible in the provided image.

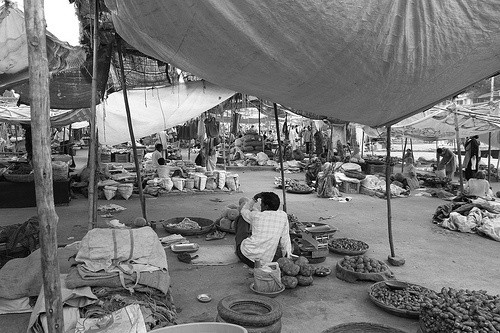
[340,181,360,193]
[102,150,144,162]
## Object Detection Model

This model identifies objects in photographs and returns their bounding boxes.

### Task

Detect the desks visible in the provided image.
[0,178,72,207]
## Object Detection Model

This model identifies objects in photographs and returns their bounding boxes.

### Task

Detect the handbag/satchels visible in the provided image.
[74,225,168,290]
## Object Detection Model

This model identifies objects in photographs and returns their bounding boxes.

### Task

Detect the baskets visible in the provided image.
[336,255,390,281]
[321,320,409,333]
[328,237,370,256]
[368,280,438,316]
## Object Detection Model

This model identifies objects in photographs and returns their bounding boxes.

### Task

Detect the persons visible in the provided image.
[169,126,177,143]
[466,172,494,201]
[250,125,330,157]
[50,125,76,167]
[235,191,291,269]
[436,147,457,180]
[404,148,414,163]
[462,135,481,180]
[197,138,221,170]
[157,158,184,179]
[152,143,171,173]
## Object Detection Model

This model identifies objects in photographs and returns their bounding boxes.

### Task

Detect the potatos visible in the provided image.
[341,255,388,274]
